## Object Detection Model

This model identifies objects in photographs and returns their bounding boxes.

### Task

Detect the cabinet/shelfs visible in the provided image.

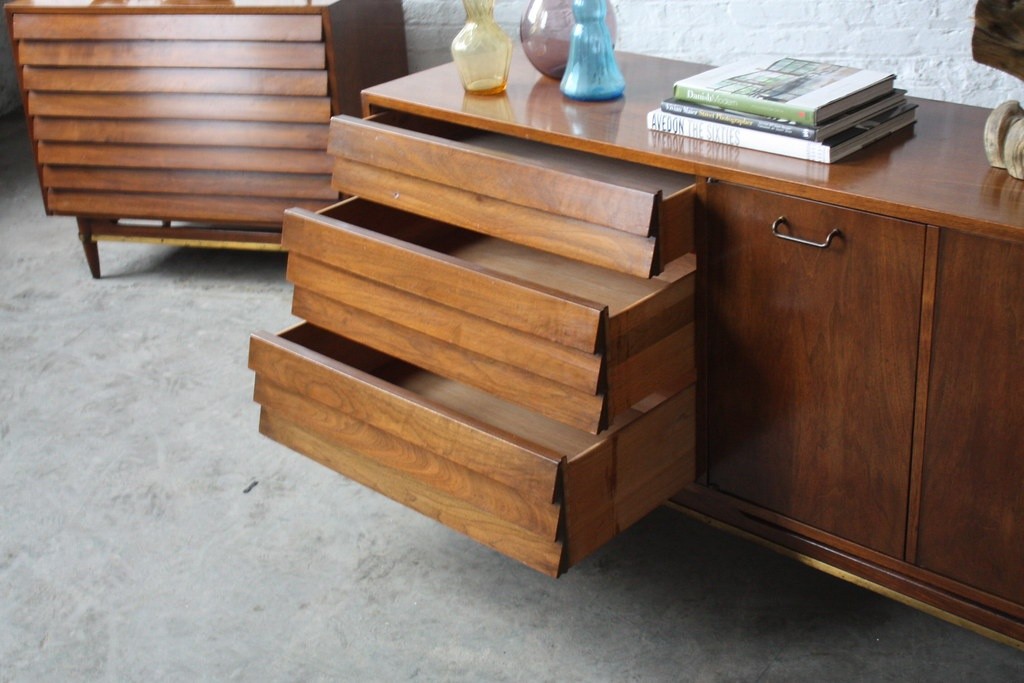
[3,0,408,281]
[248,43,1024,650]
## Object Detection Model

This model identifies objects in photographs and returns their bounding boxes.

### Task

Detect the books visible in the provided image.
[646,50,924,164]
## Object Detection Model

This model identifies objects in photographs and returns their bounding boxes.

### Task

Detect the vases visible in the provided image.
[558,0,626,100]
[520,0,617,80]
[450,0,512,94]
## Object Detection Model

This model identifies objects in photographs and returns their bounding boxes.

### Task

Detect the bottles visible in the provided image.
[451,0,625,101]
